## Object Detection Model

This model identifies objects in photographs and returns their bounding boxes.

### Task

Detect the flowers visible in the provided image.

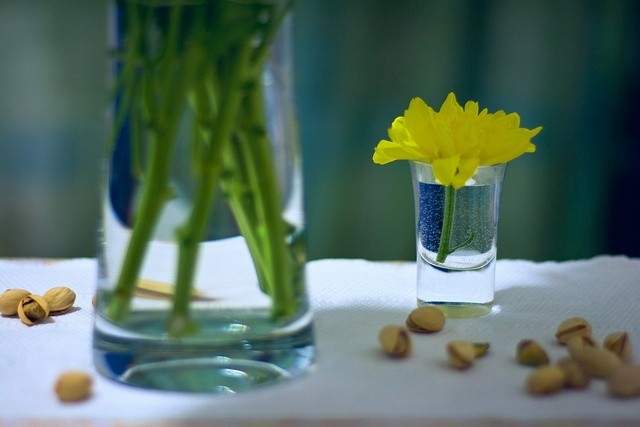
[372,92,543,263]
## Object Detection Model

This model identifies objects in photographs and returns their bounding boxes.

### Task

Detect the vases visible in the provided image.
[409,160,507,318]
[90,0,316,394]
